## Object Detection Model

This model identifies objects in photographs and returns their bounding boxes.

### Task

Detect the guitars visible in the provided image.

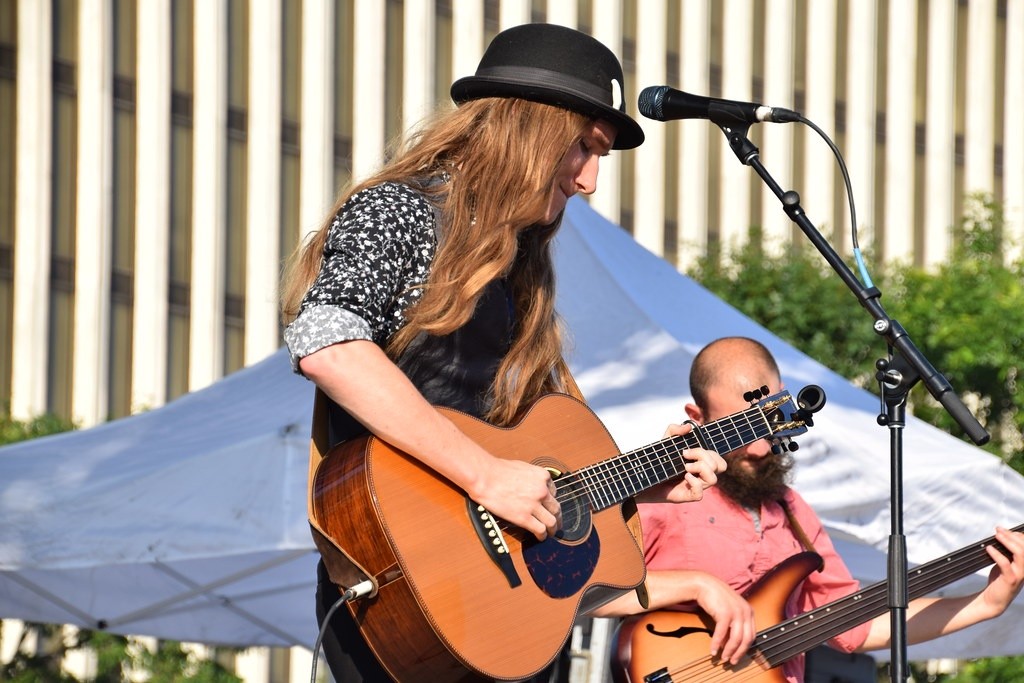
[610,523,1024,683]
[312,385,825,683]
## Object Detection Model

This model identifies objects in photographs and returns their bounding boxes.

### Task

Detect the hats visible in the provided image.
[449,22,645,151]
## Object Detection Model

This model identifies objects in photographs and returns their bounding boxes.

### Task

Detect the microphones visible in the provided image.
[637,85,800,123]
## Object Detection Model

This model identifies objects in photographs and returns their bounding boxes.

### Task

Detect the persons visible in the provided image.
[564,336,1024,683]
[276,14,732,683]
